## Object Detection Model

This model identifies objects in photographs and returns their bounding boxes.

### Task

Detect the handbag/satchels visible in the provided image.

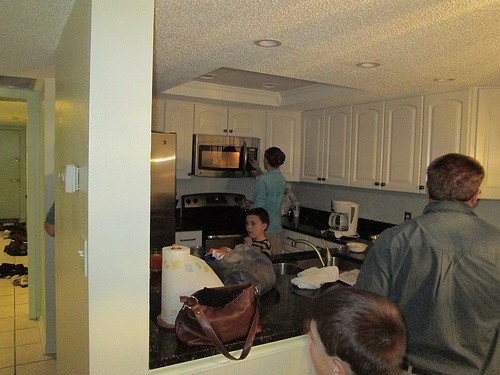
[281,183,297,216]
[175,281,259,360]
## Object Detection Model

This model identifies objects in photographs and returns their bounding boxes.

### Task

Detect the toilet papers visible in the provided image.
[160,245,225,326]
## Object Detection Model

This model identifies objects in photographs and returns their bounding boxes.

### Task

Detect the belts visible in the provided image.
[400,364,429,375]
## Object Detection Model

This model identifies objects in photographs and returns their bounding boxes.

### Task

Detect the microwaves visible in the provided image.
[192,134,261,178]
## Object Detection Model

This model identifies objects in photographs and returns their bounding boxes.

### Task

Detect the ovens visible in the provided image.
[204,235,246,250]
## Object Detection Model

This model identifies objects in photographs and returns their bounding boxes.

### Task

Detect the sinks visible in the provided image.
[295,257,361,272]
[272,263,304,276]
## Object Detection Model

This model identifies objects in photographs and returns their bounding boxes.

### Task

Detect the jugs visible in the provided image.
[329,212,348,231]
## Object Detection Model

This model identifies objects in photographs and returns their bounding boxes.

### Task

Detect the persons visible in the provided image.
[243,207,272,255]
[309,287,406,375]
[354,153,500,375]
[249,147,286,273]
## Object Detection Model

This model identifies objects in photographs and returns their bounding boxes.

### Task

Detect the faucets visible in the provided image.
[291,239,333,268]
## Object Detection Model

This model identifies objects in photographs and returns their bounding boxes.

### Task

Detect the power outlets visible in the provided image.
[404,212,412,222]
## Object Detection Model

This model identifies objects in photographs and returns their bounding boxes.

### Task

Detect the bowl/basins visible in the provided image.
[347,243,368,252]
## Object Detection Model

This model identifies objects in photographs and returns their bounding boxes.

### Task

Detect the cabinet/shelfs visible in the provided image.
[281,229,346,254]
[152,97,194,181]
[419,86,500,201]
[348,95,423,194]
[258,110,300,183]
[299,104,352,187]
[193,103,265,139]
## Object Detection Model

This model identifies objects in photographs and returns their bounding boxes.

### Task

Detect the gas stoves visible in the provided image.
[182,192,249,238]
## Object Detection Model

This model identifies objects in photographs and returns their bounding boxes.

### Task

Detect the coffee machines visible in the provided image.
[328,200,360,237]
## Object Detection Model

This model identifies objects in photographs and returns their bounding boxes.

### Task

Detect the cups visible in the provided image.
[150,248,162,273]
[293,199,300,218]
[191,245,203,259]
